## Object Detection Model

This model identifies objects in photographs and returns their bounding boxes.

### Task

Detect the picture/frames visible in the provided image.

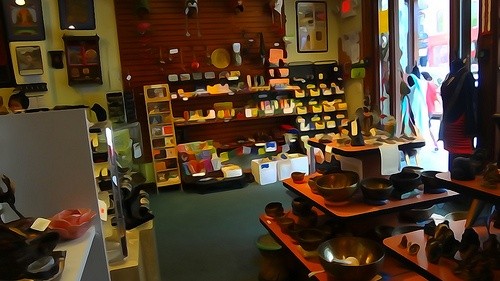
[295,0,328,53]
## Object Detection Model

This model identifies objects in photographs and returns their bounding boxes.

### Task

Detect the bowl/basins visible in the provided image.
[47,207,96,239]
[401,166,424,176]
[361,178,395,206]
[390,172,421,192]
[317,235,386,281]
[314,170,360,206]
[265,195,332,253]
[421,171,447,193]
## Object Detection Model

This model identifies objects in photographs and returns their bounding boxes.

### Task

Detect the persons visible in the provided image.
[440,58,477,156]
[400,65,442,151]
[7,93,31,114]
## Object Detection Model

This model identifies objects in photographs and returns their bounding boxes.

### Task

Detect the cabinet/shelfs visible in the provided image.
[144,83,500,281]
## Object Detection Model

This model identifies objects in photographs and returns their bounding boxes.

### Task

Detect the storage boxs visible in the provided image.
[288,153,309,177]
[272,155,291,182]
[251,158,277,185]
